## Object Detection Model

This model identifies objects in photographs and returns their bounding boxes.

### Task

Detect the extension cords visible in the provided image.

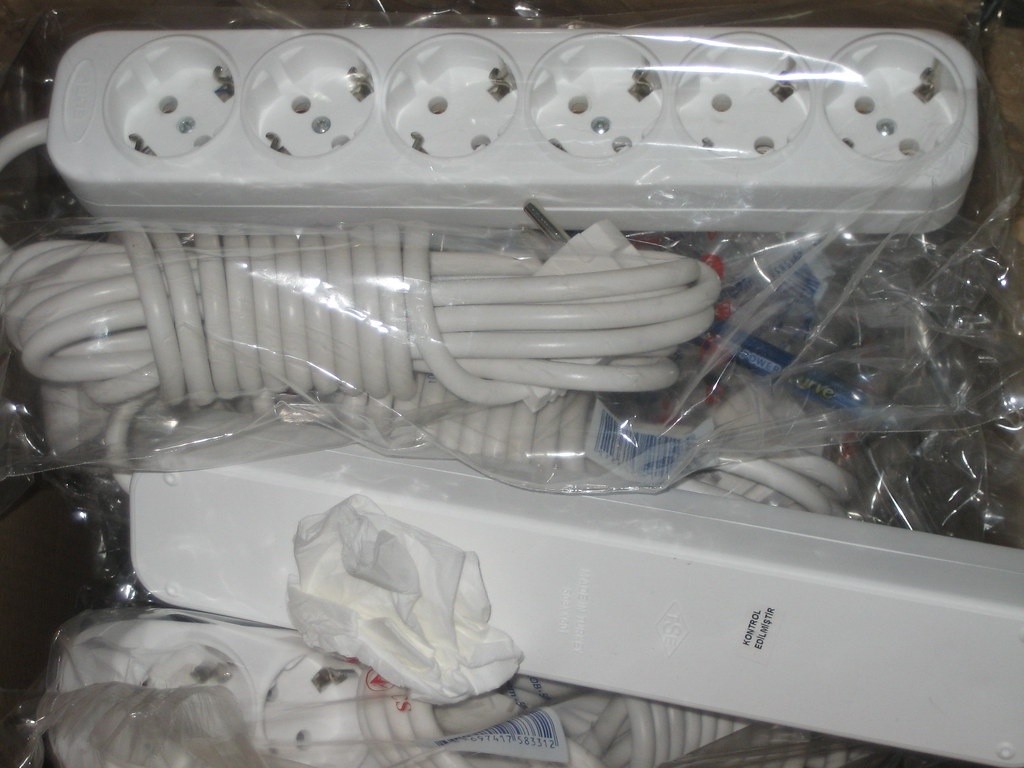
[44,407,1024,768]
[47,25,980,230]
